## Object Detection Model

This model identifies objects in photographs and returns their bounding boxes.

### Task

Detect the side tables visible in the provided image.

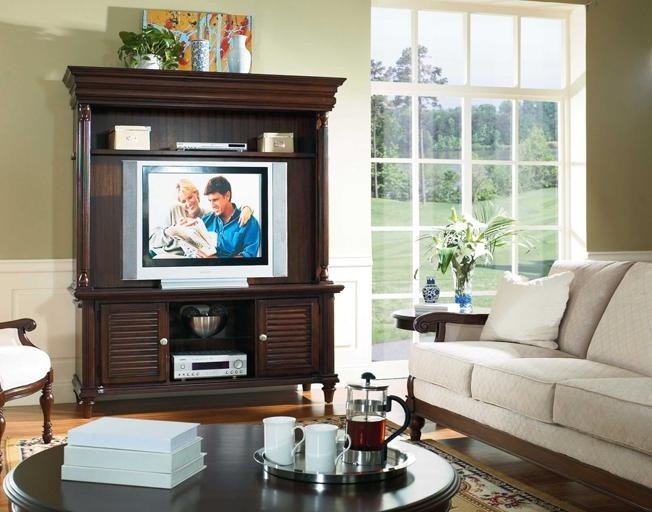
[391,303,490,331]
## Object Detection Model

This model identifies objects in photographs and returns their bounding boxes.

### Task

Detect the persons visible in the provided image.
[149,174,261,258]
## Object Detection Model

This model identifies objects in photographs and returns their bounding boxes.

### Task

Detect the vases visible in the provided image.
[227,34,252,74]
[453,271,473,314]
[422,275,440,304]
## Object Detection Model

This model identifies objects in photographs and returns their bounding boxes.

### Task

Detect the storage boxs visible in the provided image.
[257,132,295,154]
[107,124,152,151]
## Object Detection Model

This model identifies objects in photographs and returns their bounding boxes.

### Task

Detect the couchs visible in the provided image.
[404,313,652,511]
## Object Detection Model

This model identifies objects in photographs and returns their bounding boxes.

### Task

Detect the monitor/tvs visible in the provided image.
[122,160,288,289]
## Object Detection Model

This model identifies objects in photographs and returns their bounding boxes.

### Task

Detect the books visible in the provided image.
[60,415,209,489]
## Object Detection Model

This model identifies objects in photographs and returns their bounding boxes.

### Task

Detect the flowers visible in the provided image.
[413,177,548,295]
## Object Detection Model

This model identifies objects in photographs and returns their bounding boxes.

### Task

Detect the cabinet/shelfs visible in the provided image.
[69,282,345,419]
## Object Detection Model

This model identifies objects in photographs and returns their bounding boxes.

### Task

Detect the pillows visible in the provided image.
[478,270,576,351]
[585,261,652,376]
[546,259,634,359]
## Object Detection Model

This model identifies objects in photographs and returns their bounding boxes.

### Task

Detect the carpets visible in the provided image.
[4,411,582,511]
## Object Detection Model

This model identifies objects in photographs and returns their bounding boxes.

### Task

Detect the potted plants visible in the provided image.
[117,26,186,72]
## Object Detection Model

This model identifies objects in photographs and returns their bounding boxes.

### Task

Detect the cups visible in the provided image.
[305,424,352,475]
[263,416,305,466]
[343,372,409,467]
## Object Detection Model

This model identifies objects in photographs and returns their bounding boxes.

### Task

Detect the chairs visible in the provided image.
[0,317,55,473]
